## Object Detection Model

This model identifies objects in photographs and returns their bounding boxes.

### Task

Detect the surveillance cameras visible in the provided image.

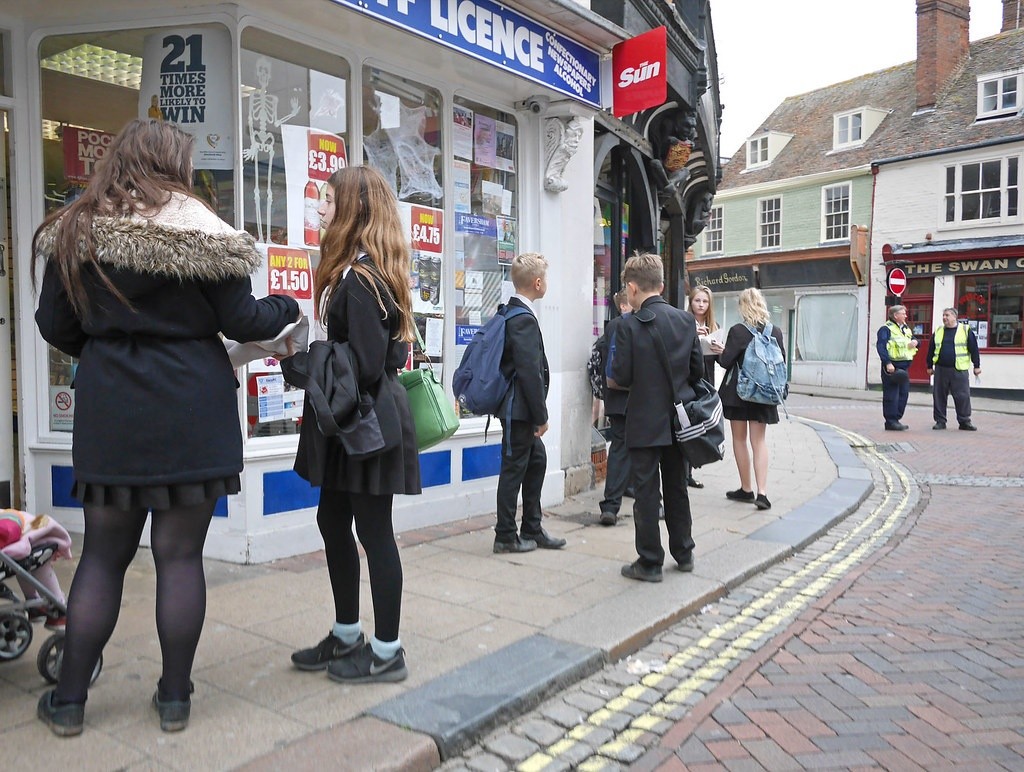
[526,96,549,115]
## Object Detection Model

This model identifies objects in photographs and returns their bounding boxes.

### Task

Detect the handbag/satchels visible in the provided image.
[391,365,460,452]
[676,377,724,468]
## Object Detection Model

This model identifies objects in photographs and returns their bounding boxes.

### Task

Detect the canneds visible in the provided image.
[410,250,442,306]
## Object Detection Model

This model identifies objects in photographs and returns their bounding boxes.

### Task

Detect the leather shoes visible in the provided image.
[933,421,947,429]
[493,531,537,553]
[520,527,566,549]
[885,420,909,430]
[959,421,977,431]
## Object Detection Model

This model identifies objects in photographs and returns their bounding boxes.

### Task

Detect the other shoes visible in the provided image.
[688,477,703,488]
[43,611,67,631]
[28,607,48,617]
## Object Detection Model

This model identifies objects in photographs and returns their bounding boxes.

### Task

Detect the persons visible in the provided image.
[926,308,981,430]
[876,305,921,430]
[709,288,786,509]
[493,253,567,554]
[30,118,304,736]
[271,165,423,684]
[148,95,162,120]
[611,252,706,582]
[684,284,721,488]
[596,289,666,525]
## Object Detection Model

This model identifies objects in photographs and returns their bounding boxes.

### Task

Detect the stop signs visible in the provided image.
[887,268,908,296]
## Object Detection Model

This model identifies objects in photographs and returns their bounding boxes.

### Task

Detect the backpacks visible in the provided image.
[587,336,604,400]
[453,303,537,415]
[732,320,789,407]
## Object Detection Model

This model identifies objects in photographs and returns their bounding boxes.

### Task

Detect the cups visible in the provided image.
[303,173,328,247]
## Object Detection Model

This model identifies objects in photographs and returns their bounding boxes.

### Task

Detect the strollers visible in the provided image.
[0,515,105,687]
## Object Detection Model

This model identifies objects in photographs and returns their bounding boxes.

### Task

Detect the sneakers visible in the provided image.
[678,552,695,571]
[38,688,87,736]
[658,505,665,519]
[623,486,636,497]
[622,558,663,582]
[599,510,616,524]
[755,494,771,508]
[152,676,193,732]
[327,641,408,684]
[726,487,754,501]
[292,630,366,671]
